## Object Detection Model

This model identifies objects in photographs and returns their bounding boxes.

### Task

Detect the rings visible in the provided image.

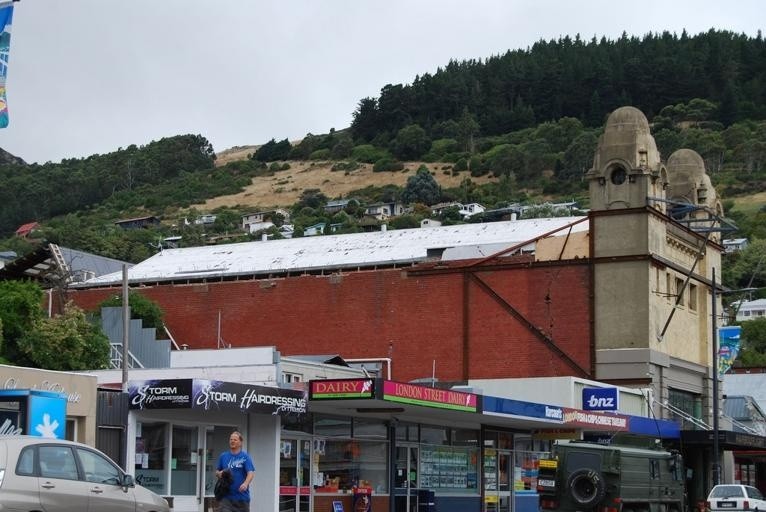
[242,486,245,488]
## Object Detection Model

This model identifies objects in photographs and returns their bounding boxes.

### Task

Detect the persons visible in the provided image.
[215,429,256,512]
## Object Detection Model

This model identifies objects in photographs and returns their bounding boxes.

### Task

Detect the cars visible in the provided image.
[0,433,171,511]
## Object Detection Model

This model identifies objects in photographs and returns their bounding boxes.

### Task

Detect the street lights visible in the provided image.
[711,268,758,484]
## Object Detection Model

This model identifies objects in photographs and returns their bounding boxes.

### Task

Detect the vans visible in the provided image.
[703,484,766,512]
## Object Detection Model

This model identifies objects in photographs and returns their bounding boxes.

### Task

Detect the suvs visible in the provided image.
[535,443,686,510]
